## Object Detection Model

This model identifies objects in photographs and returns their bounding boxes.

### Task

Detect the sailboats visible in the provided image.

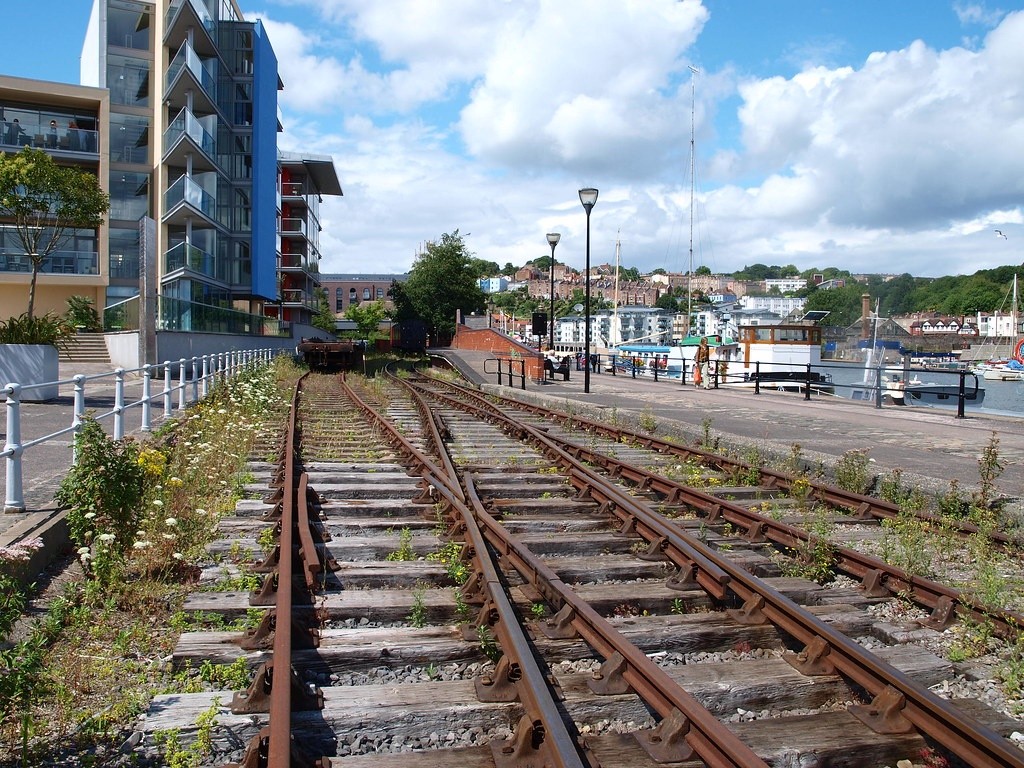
[666,68,729,379]
[969,272,1024,381]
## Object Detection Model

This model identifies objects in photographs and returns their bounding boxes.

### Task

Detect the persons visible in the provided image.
[68,121,78,150]
[48,120,57,149]
[5,118,26,146]
[694,337,710,390]
[719,362,729,383]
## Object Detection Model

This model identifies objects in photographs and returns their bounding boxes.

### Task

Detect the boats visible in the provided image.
[925,362,971,371]
[603,346,672,379]
[851,301,987,406]
[716,318,837,398]
[544,342,595,371]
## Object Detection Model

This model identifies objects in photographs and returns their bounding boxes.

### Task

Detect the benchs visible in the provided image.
[544,359,567,381]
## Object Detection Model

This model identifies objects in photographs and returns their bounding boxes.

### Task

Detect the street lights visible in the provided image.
[578,187,599,392]
[545,233,560,350]
